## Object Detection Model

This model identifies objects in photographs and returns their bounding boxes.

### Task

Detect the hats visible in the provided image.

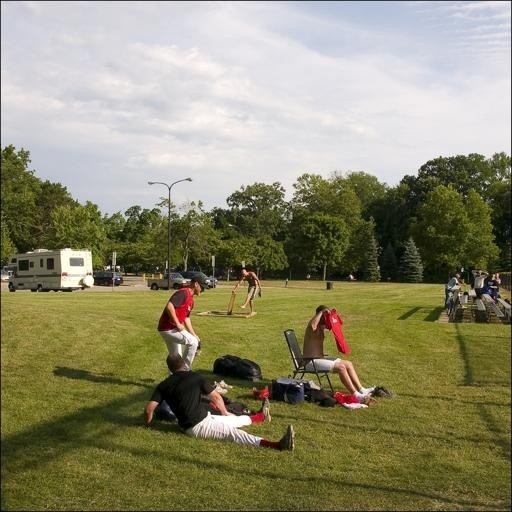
[191,275,210,290]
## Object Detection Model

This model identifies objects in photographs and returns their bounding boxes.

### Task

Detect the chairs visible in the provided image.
[283,328,335,397]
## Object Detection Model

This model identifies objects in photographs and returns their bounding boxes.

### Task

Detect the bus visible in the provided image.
[8,248,94,292]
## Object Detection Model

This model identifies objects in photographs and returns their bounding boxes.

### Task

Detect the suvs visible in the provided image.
[180,271,217,289]
[93,272,123,286]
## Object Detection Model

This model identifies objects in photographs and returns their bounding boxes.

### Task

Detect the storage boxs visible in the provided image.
[272,376,310,402]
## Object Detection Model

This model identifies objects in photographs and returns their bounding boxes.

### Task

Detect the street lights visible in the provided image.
[149,179,196,290]
[194,273,194,275]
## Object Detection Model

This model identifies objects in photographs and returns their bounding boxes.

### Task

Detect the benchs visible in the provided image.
[482,293,504,321]
[497,298,512,322]
[451,285,469,314]
[468,289,486,318]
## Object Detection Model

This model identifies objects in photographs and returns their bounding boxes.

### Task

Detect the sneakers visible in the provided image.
[279,424,294,450]
[359,393,372,401]
[365,385,377,395]
[261,396,272,422]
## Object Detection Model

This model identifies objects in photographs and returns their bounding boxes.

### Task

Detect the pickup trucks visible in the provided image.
[147,273,191,290]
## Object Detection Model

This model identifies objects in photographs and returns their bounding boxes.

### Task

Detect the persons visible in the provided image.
[233,268,261,318]
[158,273,206,371]
[471,270,488,304]
[445,274,461,308]
[303,304,376,400]
[487,274,500,303]
[494,272,500,293]
[145,351,294,452]
